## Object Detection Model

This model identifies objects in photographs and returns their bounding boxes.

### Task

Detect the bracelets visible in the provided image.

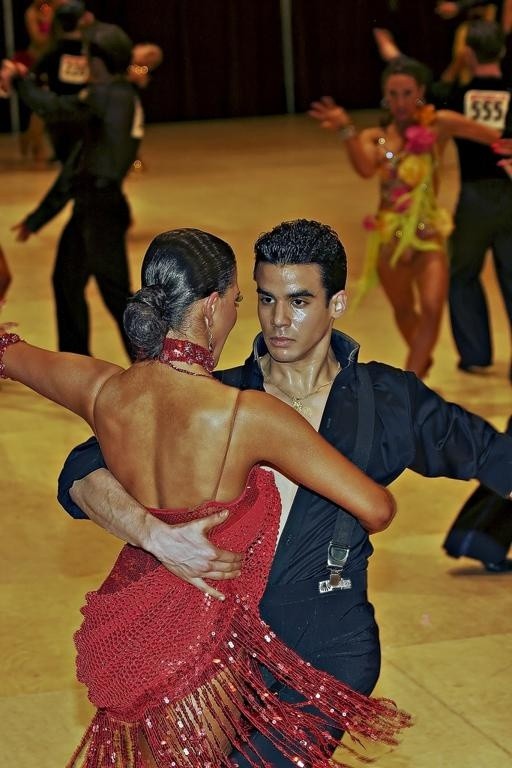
[0,333,20,381]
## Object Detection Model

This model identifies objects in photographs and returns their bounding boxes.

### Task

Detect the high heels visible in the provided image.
[442,542,512,573]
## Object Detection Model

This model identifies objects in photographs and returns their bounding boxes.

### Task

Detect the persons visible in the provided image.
[0,1,162,165]
[0,21,147,364]
[441,414,511,575]
[57,220,512,767]
[310,1,511,381]
[1,228,413,767]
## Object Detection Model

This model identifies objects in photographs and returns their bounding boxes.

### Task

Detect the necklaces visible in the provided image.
[264,356,341,412]
[136,339,221,382]
